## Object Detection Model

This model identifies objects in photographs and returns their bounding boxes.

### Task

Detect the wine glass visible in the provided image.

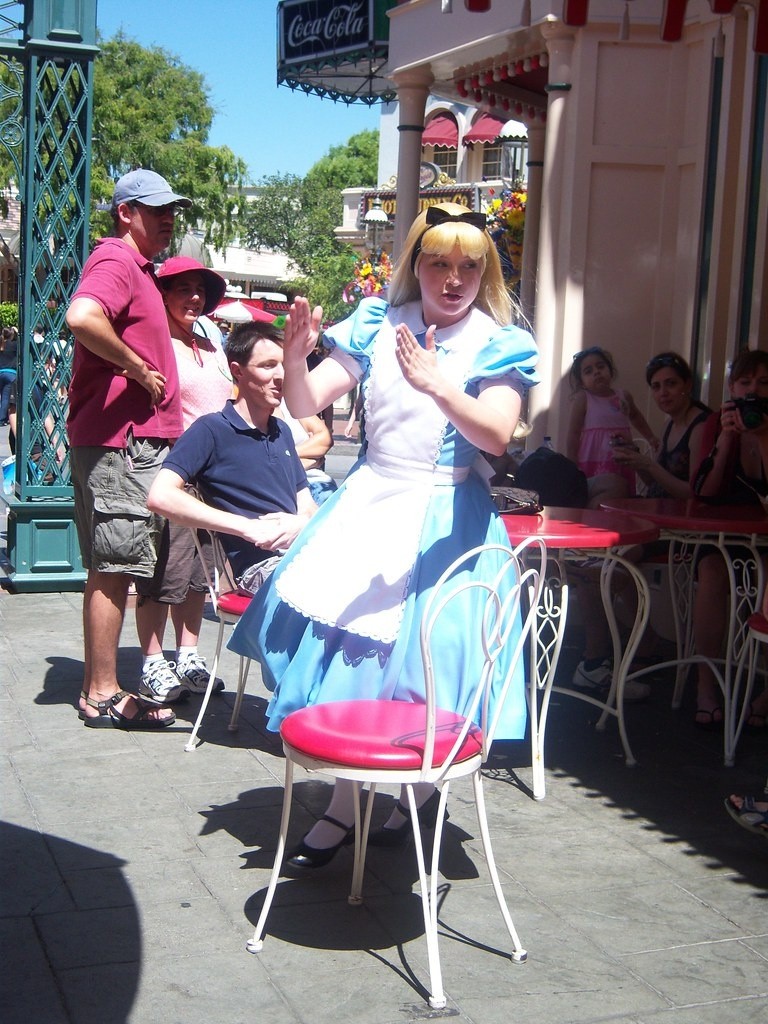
[606,439,631,465]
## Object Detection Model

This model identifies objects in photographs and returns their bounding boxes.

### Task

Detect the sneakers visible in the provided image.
[138,659,189,702]
[171,653,225,693]
[564,548,632,589]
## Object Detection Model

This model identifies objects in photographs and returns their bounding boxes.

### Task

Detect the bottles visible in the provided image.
[543,436,554,451]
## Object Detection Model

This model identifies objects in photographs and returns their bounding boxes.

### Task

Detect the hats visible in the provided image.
[113,168,193,209]
[157,256,226,316]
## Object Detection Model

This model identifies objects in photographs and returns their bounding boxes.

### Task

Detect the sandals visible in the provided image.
[725,795,768,838]
[78,688,176,729]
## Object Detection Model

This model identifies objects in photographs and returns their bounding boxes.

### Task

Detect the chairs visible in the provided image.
[181,483,254,756]
[727,611,768,760]
[241,536,551,1008]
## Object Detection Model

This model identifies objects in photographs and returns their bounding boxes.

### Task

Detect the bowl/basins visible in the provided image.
[760,497,768,513]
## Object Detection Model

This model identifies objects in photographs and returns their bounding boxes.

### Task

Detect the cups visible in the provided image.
[490,493,507,511]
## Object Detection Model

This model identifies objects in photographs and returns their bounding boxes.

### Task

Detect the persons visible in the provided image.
[612,350,717,500]
[227,201,539,871]
[142,322,319,600]
[698,350,768,498]
[0,321,74,486]
[64,166,235,733]
[566,347,661,497]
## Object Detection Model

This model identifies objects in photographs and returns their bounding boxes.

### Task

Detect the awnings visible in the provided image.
[461,113,506,146]
[421,115,458,150]
[496,120,528,139]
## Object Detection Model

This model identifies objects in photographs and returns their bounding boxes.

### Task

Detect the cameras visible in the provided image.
[725,393,768,429]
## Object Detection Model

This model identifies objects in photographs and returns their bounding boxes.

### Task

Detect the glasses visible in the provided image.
[133,204,182,217]
[575,346,600,359]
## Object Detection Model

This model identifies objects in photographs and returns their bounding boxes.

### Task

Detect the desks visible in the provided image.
[594,496,768,774]
[501,505,659,802]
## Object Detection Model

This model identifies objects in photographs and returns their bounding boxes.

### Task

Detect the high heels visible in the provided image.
[371,787,449,847]
[287,815,363,870]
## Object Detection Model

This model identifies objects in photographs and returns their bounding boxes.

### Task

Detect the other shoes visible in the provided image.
[574,658,651,701]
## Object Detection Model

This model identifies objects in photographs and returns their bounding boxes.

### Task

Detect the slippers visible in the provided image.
[737,700,768,730]
[693,684,723,731]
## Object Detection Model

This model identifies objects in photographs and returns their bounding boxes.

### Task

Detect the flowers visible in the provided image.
[482,178,529,246]
[340,249,395,306]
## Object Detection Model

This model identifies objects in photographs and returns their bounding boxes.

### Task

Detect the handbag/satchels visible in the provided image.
[488,486,543,515]
[519,447,588,508]
